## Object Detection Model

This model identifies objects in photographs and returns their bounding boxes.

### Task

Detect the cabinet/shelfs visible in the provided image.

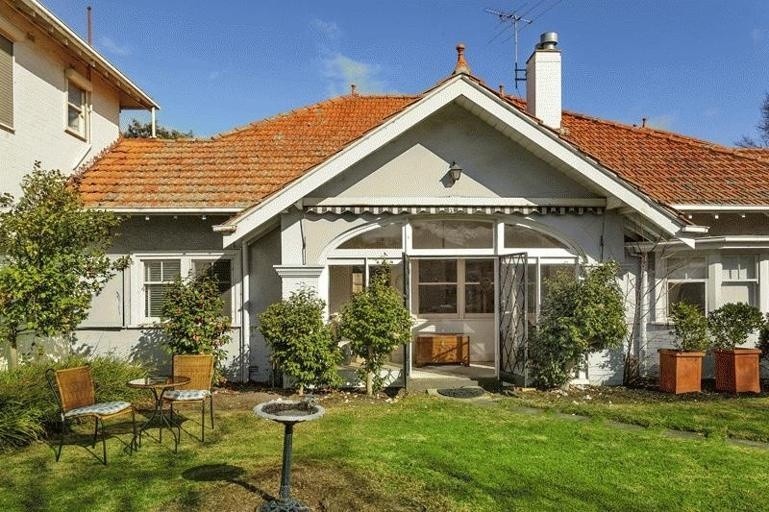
[415,333,470,368]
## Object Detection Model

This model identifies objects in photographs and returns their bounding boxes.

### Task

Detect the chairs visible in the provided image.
[45,352,219,466]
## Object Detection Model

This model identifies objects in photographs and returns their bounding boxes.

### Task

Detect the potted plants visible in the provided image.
[657,301,766,396]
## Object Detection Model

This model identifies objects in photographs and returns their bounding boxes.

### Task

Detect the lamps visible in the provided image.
[450,161,464,182]
